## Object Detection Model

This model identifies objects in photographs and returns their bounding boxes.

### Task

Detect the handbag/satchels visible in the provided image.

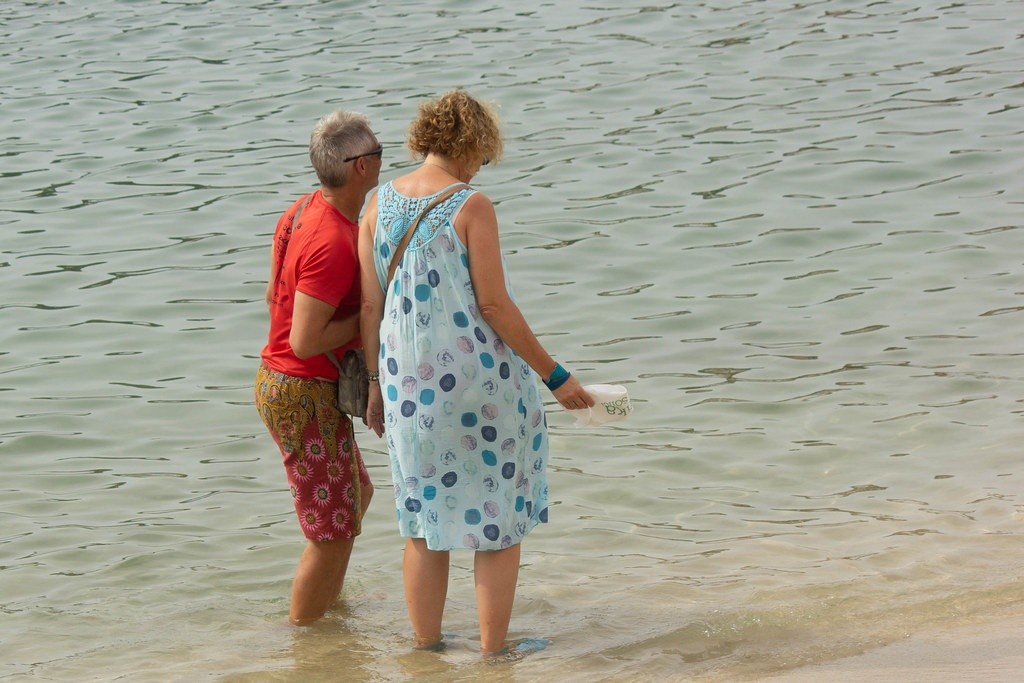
[337,349,368,416]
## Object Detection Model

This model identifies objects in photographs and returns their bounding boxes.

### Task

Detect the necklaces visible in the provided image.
[423,162,451,174]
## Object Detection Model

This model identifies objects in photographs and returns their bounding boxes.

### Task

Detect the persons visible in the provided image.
[358,94,595,656]
[254,108,383,626]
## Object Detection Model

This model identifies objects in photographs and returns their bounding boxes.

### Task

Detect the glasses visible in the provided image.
[343,143,383,163]
[482,158,490,164]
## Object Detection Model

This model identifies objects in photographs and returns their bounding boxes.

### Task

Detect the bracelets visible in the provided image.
[542,362,570,392]
[365,369,379,381]
